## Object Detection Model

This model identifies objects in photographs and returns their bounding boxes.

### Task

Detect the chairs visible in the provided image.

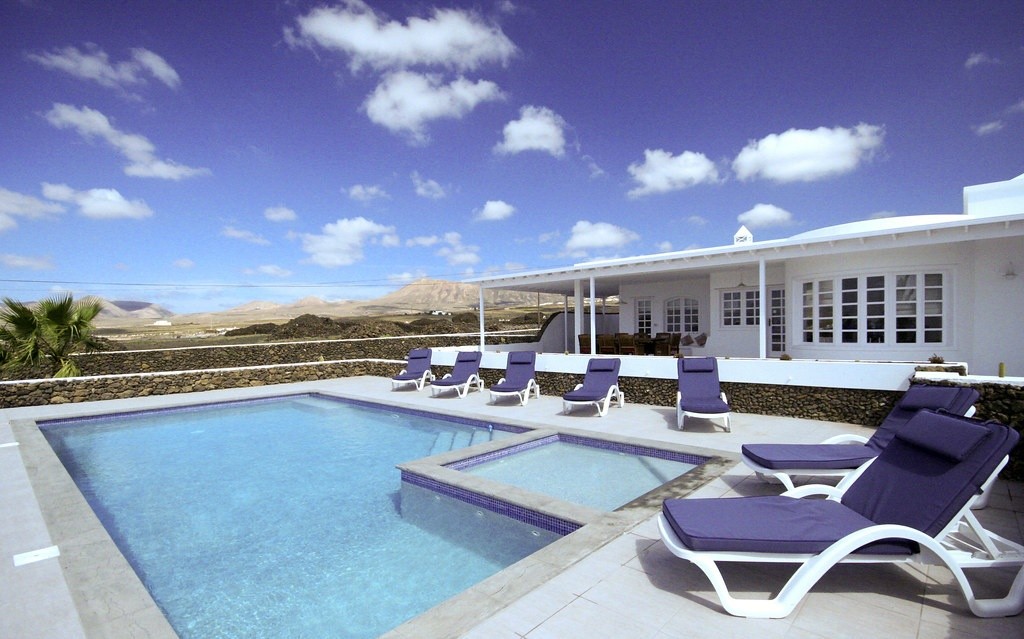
[578,332,681,356]
[561,358,625,417]
[391,349,435,392]
[490,352,540,406]
[676,356,732,433]
[657,405,1024,619]
[742,385,979,493]
[430,351,484,400]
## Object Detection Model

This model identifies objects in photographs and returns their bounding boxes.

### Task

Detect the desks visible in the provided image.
[596,338,665,345]
